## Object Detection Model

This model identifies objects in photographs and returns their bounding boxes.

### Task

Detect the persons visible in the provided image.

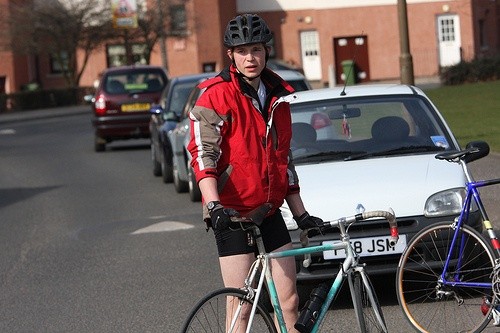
[186,14,326,333]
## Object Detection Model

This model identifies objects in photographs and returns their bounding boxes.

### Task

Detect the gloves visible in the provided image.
[294,211,326,238]
[209,204,240,231]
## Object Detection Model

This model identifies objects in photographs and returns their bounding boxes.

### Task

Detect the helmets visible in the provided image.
[223,13,272,47]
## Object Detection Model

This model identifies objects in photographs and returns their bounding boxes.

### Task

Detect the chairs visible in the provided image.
[171,87,194,111]
[290,120,321,157]
[110,79,125,93]
[145,78,162,88]
[359,117,423,152]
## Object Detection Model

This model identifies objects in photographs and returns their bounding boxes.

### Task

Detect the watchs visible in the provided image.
[207,201,220,211]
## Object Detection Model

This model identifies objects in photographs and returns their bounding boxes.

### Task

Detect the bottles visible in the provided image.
[294,284,328,333]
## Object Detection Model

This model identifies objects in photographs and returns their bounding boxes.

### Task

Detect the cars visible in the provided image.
[149,60,337,201]
[285,84,491,309]
[85,66,171,153]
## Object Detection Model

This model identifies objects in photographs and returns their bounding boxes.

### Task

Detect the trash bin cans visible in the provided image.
[341,60,356,86]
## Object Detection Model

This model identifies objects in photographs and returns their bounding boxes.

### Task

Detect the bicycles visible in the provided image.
[181,203,399,333]
[395,148,500,333]
